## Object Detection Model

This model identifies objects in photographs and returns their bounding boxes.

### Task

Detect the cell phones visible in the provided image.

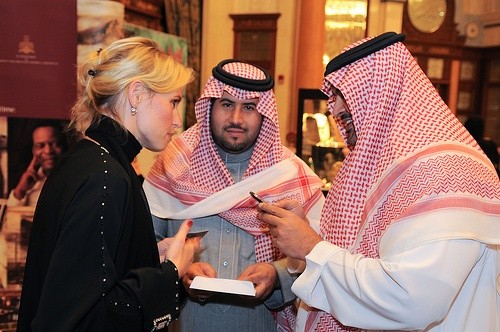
[250,191,264,204]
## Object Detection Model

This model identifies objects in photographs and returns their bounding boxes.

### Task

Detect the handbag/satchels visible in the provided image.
[311,141,347,184]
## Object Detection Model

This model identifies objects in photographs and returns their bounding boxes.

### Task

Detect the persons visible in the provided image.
[257,32,500,332]
[16,36,202,332]
[141,59,326,332]
[463,117,500,166]
[6,124,63,209]
